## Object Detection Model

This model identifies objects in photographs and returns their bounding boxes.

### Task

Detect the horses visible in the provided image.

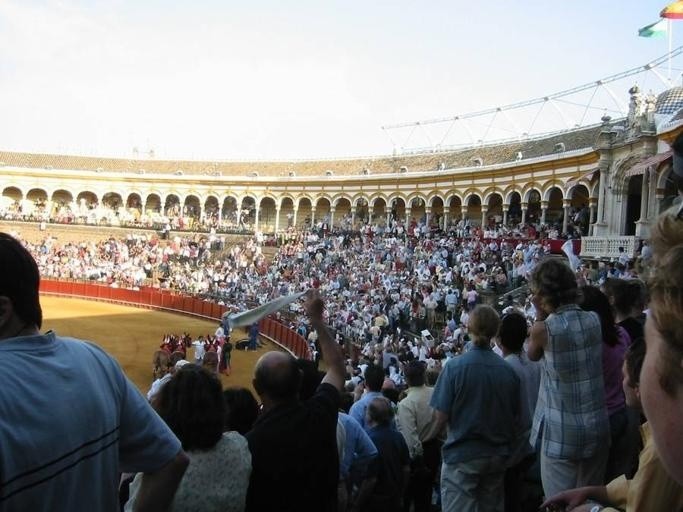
[203,335,218,373]
[170,332,191,366]
[152,333,173,384]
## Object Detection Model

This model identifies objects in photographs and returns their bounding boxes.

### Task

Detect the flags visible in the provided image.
[659,0,683,21]
[638,18,668,39]
